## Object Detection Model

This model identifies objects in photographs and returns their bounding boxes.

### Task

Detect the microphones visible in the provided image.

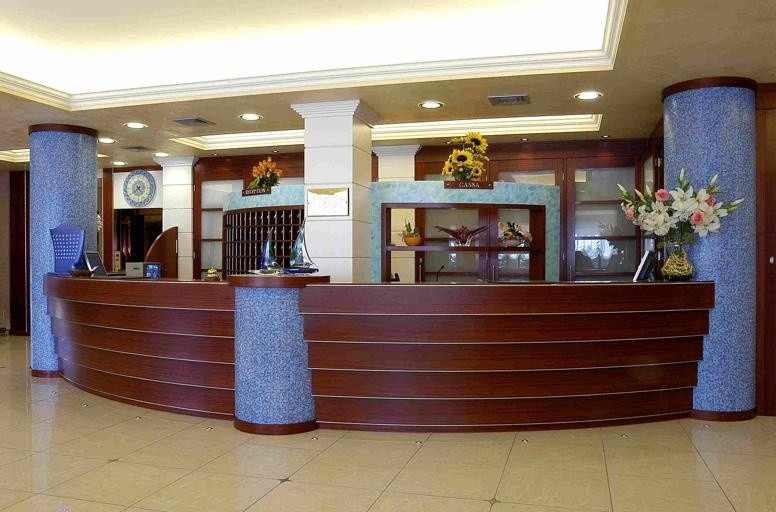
[437,264,444,281]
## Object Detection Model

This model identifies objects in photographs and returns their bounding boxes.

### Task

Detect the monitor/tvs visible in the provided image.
[632,250,657,281]
[83,250,108,279]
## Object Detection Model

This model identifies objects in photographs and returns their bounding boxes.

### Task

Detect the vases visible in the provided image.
[660,242,694,281]
[502,239,521,247]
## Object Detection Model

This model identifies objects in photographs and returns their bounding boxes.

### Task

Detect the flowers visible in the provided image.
[440,130,490,181]
[251,156,282,186]
[497,221,533,243]
[617,168,745,254]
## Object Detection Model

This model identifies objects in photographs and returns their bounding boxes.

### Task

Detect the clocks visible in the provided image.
[123,169,156,208]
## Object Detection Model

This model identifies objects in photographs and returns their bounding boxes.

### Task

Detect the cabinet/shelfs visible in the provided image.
[381,202,546,284]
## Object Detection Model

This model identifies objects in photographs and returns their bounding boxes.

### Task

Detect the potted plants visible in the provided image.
[402,221,423,246]
